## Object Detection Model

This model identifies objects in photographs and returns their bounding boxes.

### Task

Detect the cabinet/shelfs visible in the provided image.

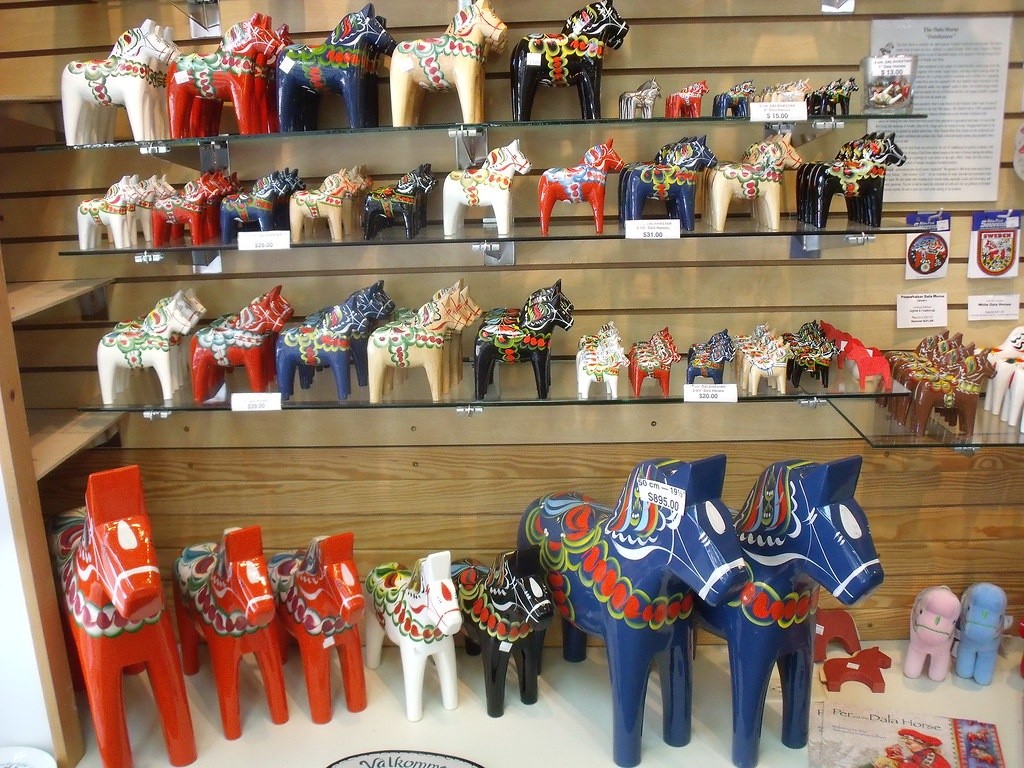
[0,0,1024,487]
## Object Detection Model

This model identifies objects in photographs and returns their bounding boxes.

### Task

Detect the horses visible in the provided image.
[90,282,1022,443]
[72,131,905,250]
[53,452,1017,768]
[58,0,914,145]
